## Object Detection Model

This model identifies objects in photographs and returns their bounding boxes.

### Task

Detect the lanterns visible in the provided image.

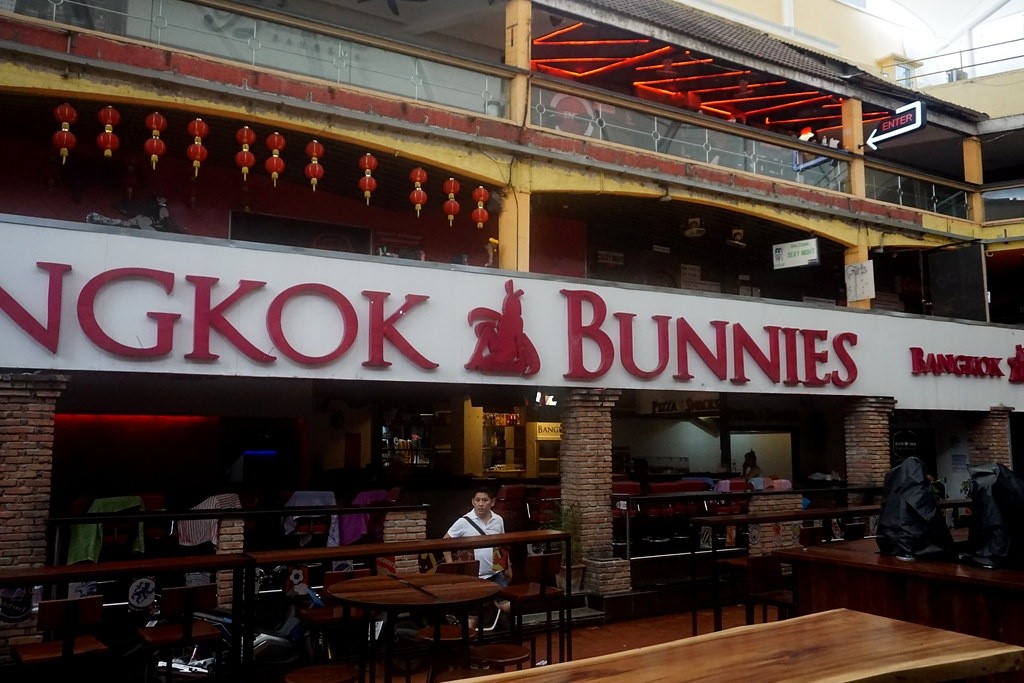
[359,154,378,205]
[409,169,489,228]
[53,101,325,192]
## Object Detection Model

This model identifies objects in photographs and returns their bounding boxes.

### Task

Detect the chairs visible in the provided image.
[691,545,795,637]
[137,582,220,682]
[60,491,398,560]
[13,595,109,682]
[280,551,565,683]
[492,477,790,531]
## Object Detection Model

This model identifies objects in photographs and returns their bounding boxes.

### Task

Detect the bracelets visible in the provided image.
[508,563,512,567]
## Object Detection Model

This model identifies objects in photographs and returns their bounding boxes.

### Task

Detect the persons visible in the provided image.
[742,451,761,483]
[442,487,512,628]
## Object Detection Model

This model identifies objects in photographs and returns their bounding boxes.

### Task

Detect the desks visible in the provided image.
[326,572,502,683]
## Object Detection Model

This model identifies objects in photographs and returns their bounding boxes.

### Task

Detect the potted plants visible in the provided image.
[540,502,585,592]
